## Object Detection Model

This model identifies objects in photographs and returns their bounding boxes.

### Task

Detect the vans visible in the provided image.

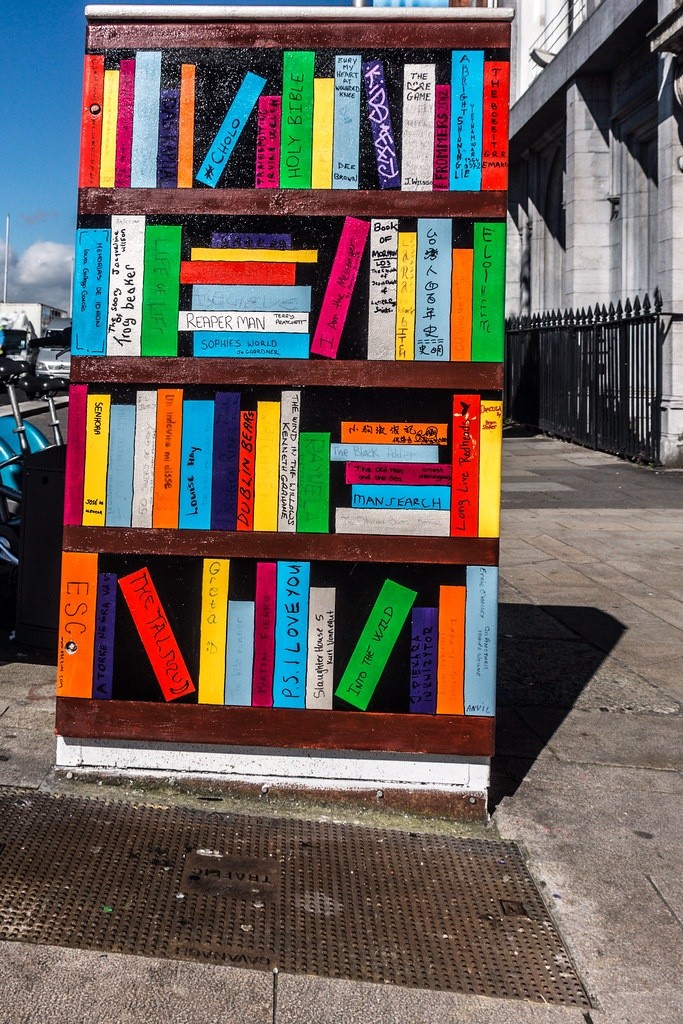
[0,328,36,400]
[35,318,72,391]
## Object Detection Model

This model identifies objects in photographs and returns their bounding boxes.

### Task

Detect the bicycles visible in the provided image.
[1,374,69,629]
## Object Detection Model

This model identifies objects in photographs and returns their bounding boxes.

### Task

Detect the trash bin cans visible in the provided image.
[14,446,66,665]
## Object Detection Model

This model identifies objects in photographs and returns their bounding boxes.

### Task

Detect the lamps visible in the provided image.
[530,48,557,68]
